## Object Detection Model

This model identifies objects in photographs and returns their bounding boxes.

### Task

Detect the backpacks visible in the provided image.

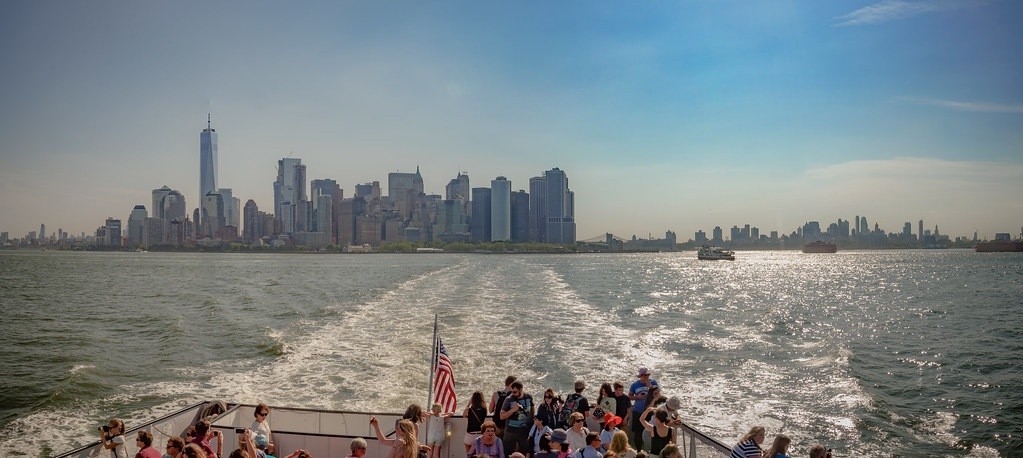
[565,393,586,426]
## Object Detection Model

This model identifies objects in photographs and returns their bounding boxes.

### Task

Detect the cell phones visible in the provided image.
[214,432,218,436]
[234,428,245,434]
[370,418,374,425]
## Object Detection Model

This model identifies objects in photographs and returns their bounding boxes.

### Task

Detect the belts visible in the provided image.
[468,432,482,435]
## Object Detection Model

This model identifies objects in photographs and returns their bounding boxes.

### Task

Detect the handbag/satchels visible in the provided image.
[593,408,605,420]
[528,434,536,450]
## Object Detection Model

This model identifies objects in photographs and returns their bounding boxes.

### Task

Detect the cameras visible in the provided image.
[98,425,110,432]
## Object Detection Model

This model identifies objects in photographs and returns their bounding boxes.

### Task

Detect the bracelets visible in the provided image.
[466,406,469,410]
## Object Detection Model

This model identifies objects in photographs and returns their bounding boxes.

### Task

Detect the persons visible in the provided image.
[425,403,454,458]
[810,445,833,458]
[729,426,768,458]
[462,367,688,458]
[370,402,431,458]
[228,401,313,458]
[165,413,223,458]
[765,433,793,458]
[346,437,367,458]
[134,431,163,458]
[98,418,129,458]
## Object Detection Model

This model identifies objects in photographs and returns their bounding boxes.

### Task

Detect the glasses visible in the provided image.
[510,390,518,395]
[573,419,585,423]
[358,447,367,451]
[108,427,116,430]
[186,432,193,437]
[182,450,187,455]
[595,439,602,442]
[259,413,268,417]
[166,444,176,449]
[543,395,552,400]
[136,438,143,442]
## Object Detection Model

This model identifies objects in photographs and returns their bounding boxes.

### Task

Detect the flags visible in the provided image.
[434,334,457,413]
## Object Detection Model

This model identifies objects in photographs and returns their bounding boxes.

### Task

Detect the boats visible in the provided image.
[802,241,838,253]
[976,238,1023,252]
[697,244,735,260]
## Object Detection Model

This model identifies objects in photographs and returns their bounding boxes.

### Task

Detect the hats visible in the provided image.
[574,380,588,389]
[431,404,442,411]
[636,367,652,378]
[544,429,572,445]
[603,412,623,427]
[665,396,682,410]
